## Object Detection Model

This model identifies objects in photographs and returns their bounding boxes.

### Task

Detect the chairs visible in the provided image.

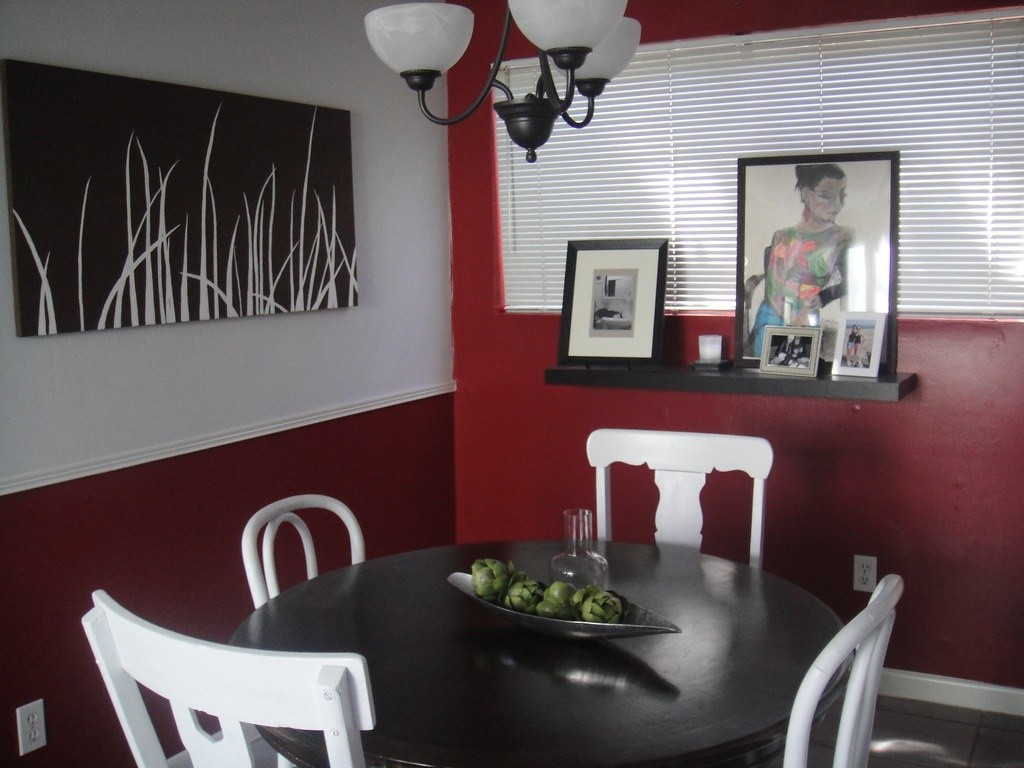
[241,494,366,768]
[81,589,377,768]
[587,428,774,570]
[782,574,904,768]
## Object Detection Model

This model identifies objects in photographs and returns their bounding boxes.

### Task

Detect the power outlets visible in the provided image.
[16,698,47,756]
[853,554,878,594]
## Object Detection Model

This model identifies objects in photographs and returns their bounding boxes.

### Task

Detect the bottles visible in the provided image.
[548,509,609,591]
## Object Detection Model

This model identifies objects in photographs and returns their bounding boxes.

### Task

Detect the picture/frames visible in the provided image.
[760,325,823,378]
[831,312,887,377]
[734,152,900,374]
[557,238,669,366]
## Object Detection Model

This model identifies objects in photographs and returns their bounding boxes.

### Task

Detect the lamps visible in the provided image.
[363,0,641,163]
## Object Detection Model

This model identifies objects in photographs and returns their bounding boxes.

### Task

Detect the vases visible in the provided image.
[548,508,608,590]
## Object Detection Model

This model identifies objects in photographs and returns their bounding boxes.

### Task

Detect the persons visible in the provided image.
[854,325,865,367]
[787,336,807,363]
[775,336,794,366]
[846,324,857,366]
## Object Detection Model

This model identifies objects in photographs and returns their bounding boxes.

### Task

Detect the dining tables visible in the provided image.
[227,538,855,768]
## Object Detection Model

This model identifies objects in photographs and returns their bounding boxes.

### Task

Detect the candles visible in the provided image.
[698,334,722,363]
